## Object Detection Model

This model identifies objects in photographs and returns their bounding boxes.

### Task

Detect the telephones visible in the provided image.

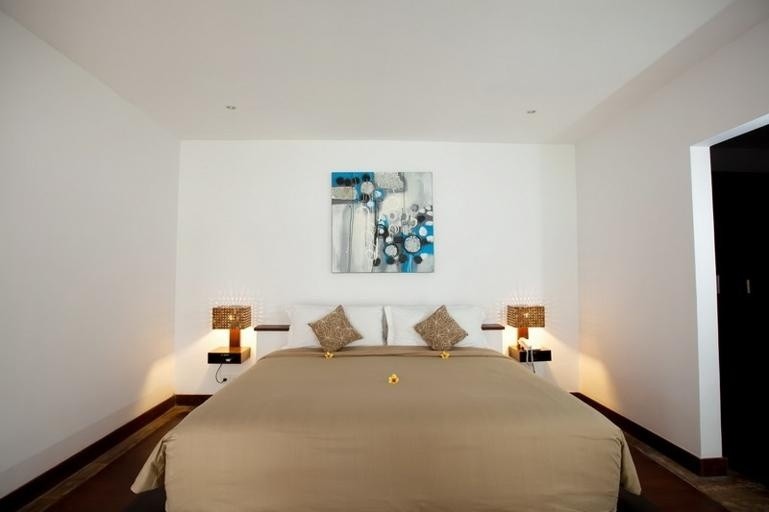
[518,337,540,351]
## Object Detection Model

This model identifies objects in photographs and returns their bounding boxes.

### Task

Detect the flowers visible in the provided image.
[439,350,449,360]
[323,350,334,360]
[387,373,400,385]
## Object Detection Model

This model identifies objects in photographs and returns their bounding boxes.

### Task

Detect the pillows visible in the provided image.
[283,303,486,355]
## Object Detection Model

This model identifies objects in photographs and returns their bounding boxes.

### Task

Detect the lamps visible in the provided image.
[211,303,252,350]
[505,303,547,347]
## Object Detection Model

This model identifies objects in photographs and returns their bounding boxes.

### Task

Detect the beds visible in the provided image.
[158,322,624,510]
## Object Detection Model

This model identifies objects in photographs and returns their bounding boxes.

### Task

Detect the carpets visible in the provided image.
[46,408,188,511]
[618,430,729,511]
